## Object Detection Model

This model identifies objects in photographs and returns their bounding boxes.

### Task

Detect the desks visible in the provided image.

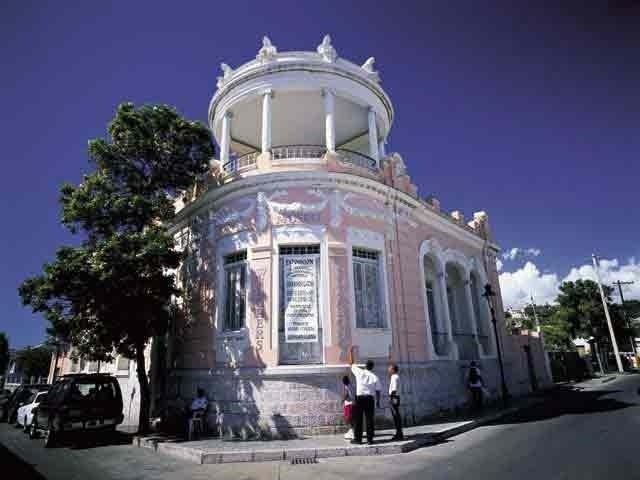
[482,284,506,394]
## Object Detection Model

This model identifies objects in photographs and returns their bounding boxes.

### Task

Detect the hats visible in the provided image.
[1,371,123,449]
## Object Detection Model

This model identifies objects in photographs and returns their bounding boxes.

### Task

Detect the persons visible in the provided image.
[349,346,382,445]
[342,374,355,439]
[387,364,405,441]
[464,361,485,419]
[584,355,594,377]
[175,388,209,438]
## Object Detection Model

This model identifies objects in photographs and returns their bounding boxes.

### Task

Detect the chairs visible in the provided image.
[350,437,374,445]
[392,433,404,441]
[344,431,353,438]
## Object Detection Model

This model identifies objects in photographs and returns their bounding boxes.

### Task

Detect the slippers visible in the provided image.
[469,368,479,384]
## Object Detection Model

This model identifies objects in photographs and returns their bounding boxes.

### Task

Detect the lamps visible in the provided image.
[192,408,209,436]
